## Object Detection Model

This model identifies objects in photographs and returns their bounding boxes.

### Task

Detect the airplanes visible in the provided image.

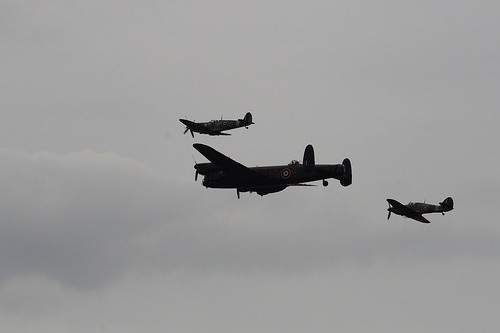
[189,141,353,198]
[386,193,455,226]
[180,112,255,139]
